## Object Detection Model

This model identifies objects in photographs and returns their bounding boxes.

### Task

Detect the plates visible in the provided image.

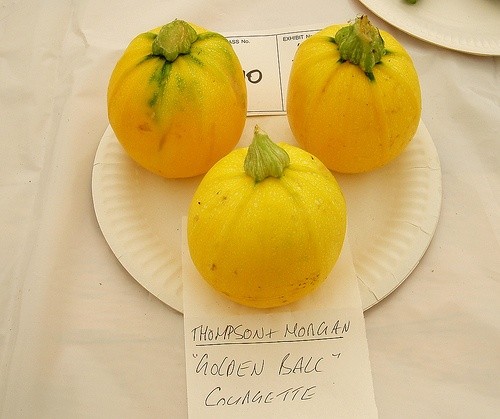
[356,1,500,57]
[91,102,445,322]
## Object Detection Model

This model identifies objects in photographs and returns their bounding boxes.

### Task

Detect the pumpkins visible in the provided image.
[105,16,248,180]
[189,123,349,310]
[286,12,423,174]
